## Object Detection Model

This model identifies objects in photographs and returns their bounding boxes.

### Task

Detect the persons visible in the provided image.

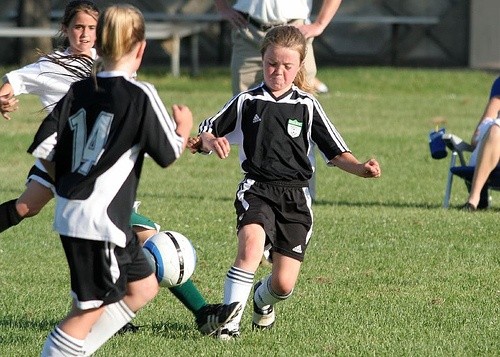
[0,0,137,232]
[197,26,381,341]
[214,0,341,204]
[461,76,500,212]
[24,1,244,357]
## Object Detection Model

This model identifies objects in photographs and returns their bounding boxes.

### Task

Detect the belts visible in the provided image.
[237,9,297,32]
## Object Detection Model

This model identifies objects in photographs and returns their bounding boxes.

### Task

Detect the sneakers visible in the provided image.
[197,301,242,338]
[214,328,239,342]
[252,280,275,332]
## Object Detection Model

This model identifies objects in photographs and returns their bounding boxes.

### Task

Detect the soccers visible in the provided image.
[142,229,196,288]
